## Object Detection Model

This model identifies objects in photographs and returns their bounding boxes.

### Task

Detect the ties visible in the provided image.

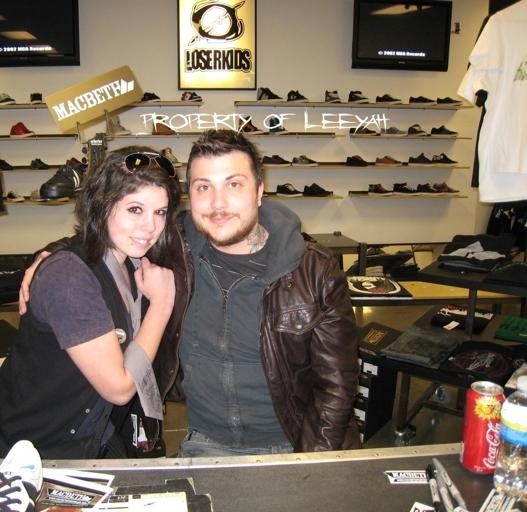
[102,243,163,419]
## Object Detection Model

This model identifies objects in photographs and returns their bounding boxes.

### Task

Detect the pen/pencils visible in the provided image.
[426,455,466,511]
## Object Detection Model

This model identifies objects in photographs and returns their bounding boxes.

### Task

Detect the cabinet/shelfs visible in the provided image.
[356,255,527,443]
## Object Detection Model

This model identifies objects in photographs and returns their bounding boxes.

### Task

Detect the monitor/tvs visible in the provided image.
[351,0,452,71]
[0,0,80,67]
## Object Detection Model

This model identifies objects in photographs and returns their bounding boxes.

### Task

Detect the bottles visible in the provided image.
[494,376,527,498]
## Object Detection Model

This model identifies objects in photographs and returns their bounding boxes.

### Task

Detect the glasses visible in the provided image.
[124,151,176,178]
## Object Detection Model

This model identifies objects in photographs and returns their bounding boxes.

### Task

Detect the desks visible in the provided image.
[308,230,368,276]
[2,439,527,511]
[344,275,527,339]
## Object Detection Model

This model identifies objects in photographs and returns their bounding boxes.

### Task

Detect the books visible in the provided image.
[380,325,459,365]
[386,338,459,369]
[345,275,413,301]
[358,321,403,356]
[493,314,527,344]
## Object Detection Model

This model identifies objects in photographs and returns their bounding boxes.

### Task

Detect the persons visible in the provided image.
[0,146,182,459]
[16,127,363,458]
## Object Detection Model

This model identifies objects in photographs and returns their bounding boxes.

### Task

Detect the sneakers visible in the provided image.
[437,97,463,105]
[257,88,288,102]
[0,93,15,105]
[433,154,458,164]
[434,182,460,193]
[31,93,42,104]
[408,152,433,165]
[238,116,264,134]
[288,90,309,102]
[375,156,402,167]
[181,92,202,102]
[347,155,370,166]
[348,91,368,103]
[153,117,175,135]
[350,126,375,135]
[368,184,393,195]
[270,118,289,134]
[325,90,341,103]
[410,96,437,105]
[303,183,333,196]
[0,160,13,170]
[0,439,44,512]
[10,123,35,137]
[162,148,182,167]
[31,159,49,169]
[292,156,318,167]
[392,183,417,193]
[6,191,25,202]
[376,95,402,104]
[106,117,130,136]
[276,184,303,197]
[381,127,407,136]
[263,156,291,167]
[408,124,430,136]
[417,184,438,193]
[142,92,160,102]
[40,158,88,198]
[30,191,48,202]
[431,126,458,137]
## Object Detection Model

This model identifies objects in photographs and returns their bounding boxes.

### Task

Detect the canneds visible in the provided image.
[458,381,505,475]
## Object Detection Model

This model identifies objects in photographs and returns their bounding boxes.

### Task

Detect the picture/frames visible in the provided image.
[175,0,259,92]
